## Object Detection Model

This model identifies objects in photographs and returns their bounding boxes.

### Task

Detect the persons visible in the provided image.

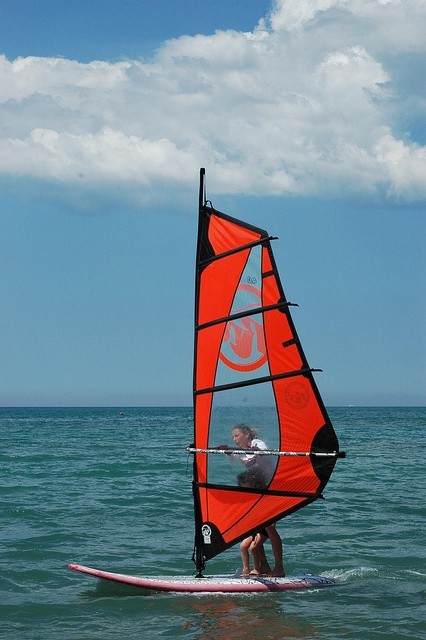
[218,423,286,578]
[235,466,269,576]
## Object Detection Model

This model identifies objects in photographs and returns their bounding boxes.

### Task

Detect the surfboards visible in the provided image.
[68,563,334,591]
